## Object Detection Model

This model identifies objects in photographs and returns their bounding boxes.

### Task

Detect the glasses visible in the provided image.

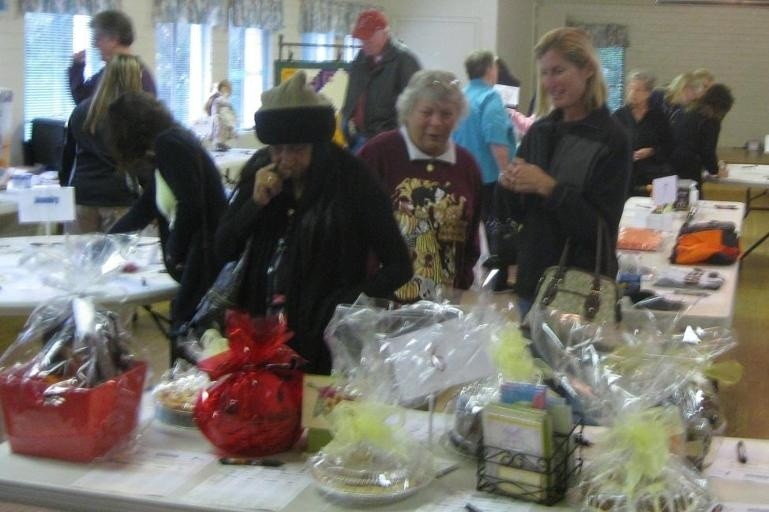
[430,79,462,96]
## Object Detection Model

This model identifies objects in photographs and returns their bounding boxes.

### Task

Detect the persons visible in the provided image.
[333,7,422,159]
[612,69,740,198]
[209,69,416,376]
[55,53,146,238]
[66,9,159,105]
[104,89,231,346]
[350,27,628,317]
[203,78,240,154]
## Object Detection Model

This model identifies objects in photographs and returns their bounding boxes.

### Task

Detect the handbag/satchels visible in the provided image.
[670,221,739,265]
[520,265,623,330]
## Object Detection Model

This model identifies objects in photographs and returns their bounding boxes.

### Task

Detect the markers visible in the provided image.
[737,440,747,463]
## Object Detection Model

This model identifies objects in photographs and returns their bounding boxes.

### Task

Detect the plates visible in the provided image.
[305,466,435,502]
[151,379,194,415]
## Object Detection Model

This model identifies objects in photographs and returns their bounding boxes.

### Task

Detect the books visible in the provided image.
[478,379,576,505]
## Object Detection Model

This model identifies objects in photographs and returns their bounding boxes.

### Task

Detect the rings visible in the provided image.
[268,176,272,183]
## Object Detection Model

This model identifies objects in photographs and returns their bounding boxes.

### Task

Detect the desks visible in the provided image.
[702,163,769,260]
[1,175,65,235]
[0,235,182,379]
[607,194,747,393]
[204,148,258,193]
[0,371,769,512]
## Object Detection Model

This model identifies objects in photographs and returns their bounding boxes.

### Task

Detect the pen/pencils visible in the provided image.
[221,457,286,466]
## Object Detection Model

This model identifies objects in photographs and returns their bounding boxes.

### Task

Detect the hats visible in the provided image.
[254,69,335,144]
[352,11,386,38]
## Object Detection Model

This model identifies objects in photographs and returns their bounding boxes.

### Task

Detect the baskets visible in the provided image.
[475,411,583,506]
